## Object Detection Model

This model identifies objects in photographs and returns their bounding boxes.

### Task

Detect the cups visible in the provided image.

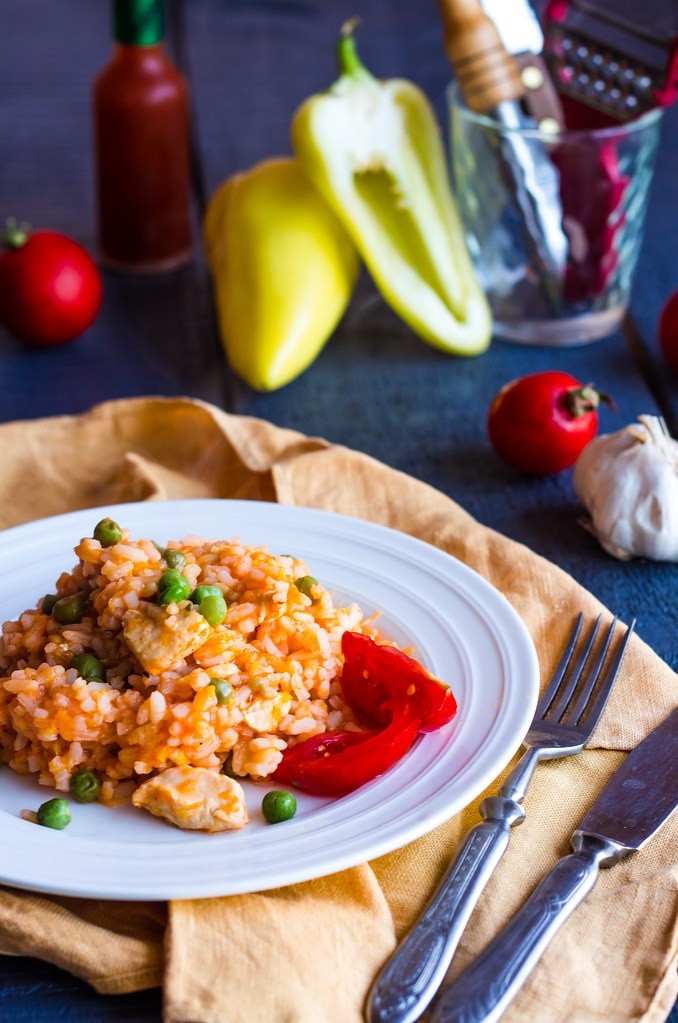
[435,35,659,349]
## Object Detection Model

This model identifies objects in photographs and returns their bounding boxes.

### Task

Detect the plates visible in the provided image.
[2,498,540,905]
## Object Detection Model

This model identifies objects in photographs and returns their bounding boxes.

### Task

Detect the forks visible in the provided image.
[358,611,641,1023]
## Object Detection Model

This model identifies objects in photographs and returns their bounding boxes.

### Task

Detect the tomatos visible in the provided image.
[0,215,102,352]
[489,368,619,480]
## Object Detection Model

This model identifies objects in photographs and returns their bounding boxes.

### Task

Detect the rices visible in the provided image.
[0,528,418,829]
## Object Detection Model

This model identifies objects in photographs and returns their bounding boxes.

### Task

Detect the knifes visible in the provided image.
[423,692,678,1023]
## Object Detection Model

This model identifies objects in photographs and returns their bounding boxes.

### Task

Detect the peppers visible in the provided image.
[202,154,363,395]
[287,16,495,356]
[274,630,459,793]
